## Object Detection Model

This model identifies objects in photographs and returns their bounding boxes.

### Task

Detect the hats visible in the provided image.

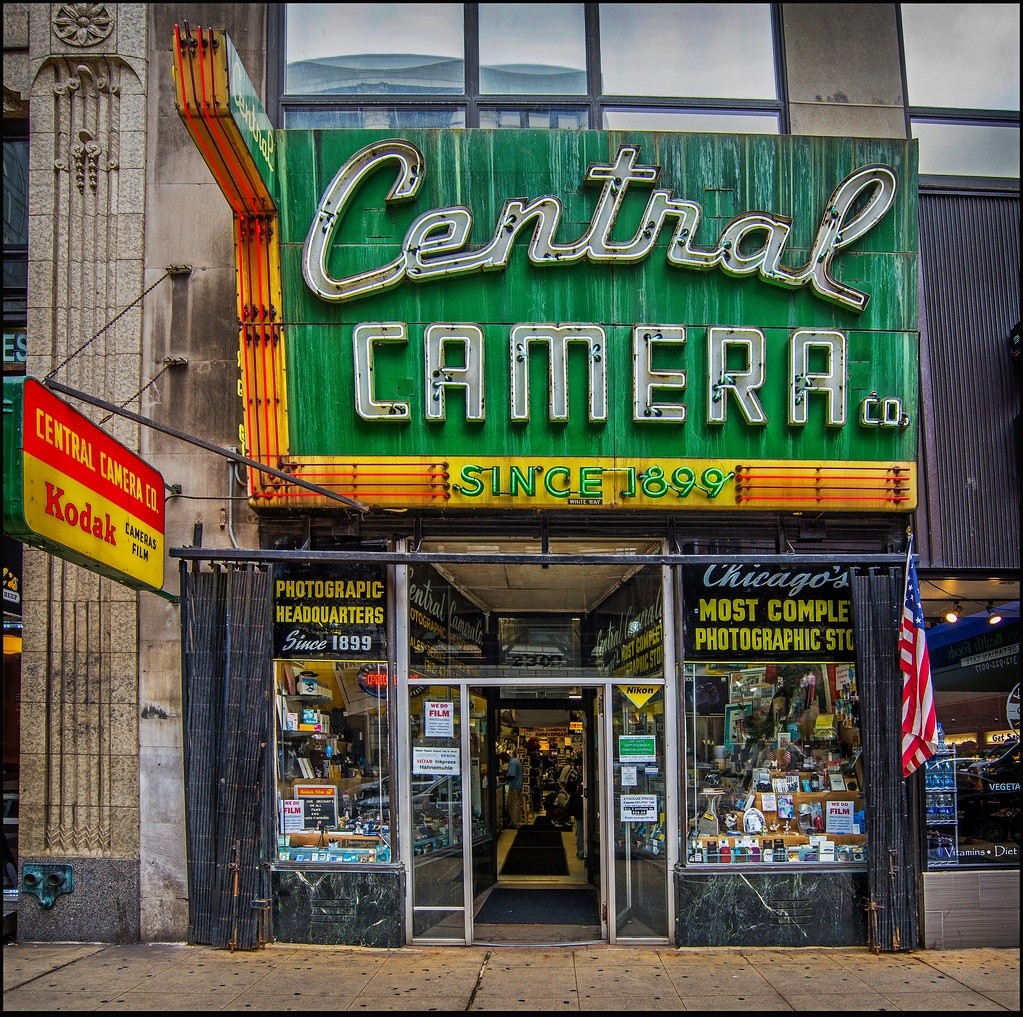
[566,780,576,793]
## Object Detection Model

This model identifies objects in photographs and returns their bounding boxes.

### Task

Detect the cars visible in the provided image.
[353,771,462,825]
[929,756,983,792]
[964,740,1022,845]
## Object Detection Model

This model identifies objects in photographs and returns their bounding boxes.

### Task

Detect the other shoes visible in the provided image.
[506,823,517,828]
[560,820,572,827]
[551,819,564,828]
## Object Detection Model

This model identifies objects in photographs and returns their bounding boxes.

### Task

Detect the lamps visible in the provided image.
[985,604,1001,624]
[945,606,964,624]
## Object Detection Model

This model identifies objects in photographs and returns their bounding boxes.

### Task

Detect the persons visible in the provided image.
[499,751,524,828]
[543,782,577,827]
[956,740,982,845]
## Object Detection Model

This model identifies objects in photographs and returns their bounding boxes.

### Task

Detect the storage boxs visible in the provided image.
[298,757,309,779]
[285,708,330,734]
[303,758,316,779]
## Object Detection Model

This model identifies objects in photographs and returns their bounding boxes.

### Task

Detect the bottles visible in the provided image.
[810,772,819,792]
[815,813,823,833]
[926,722,955,863]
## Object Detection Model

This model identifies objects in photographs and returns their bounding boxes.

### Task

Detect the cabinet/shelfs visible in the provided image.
[282,694,333,738]
[925,741,959,865]
[482,784,506,838]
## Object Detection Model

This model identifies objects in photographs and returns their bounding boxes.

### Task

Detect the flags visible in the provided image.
[897,536,939,779]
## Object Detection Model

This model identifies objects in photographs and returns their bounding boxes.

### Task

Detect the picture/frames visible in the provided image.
[728,671,763,703]
[723,700,753,752]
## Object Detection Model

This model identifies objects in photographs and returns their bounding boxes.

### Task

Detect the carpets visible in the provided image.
[474,888,601,926]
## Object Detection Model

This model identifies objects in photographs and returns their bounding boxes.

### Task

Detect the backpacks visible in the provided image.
[543,791,568,811]
